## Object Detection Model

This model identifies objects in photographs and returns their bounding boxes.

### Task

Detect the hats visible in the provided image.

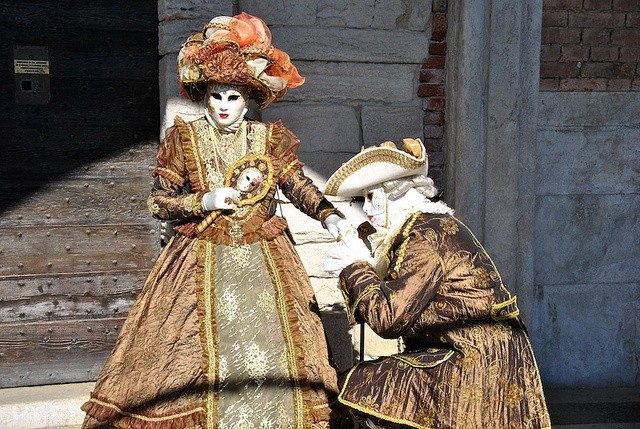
[323,137,437,197]
[177,12,306,111]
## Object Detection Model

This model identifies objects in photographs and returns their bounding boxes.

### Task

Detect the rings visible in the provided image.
[337,234,343,242]
[224,197,233,205]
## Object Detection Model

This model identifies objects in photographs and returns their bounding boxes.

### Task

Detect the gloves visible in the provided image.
[202,186,240,211]
[325,213,350,240]
[340,230,369,269]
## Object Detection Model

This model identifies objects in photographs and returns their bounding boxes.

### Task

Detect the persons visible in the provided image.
[78,12,356,428]
[325,137,552,429]
